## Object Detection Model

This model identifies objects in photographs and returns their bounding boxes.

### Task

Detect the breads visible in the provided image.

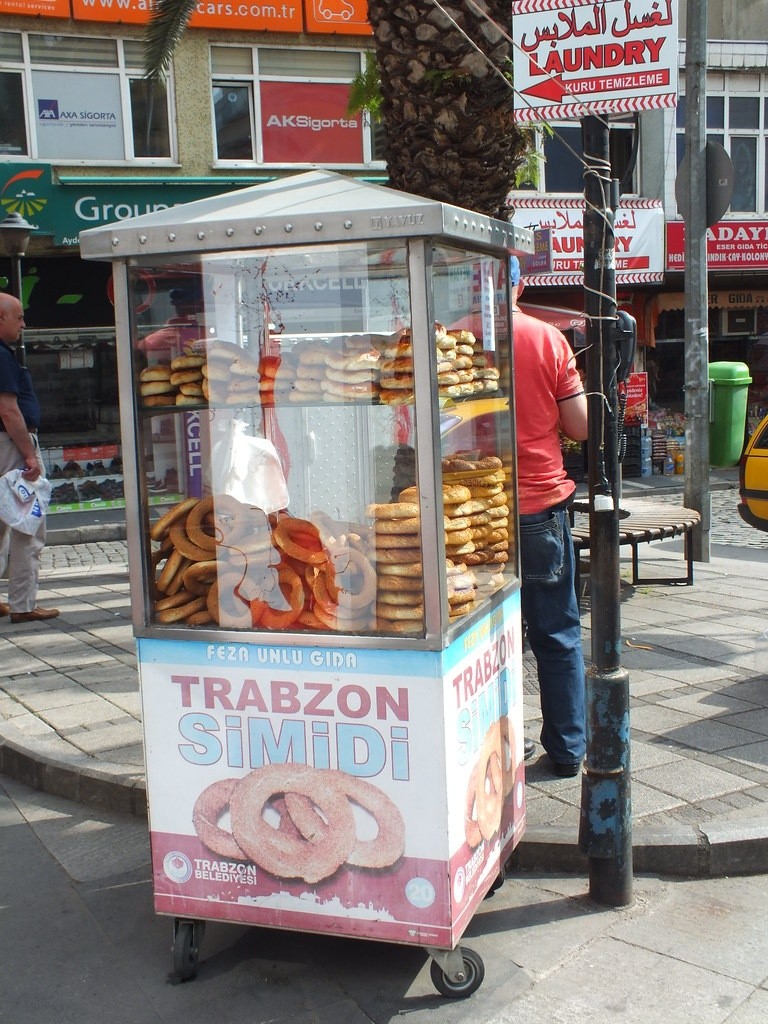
[141,320,501,406]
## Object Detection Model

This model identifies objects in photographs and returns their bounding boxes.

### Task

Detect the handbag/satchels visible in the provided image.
[0,467,52,535]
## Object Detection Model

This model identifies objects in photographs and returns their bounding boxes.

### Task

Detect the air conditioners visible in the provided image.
[718,308,758,336]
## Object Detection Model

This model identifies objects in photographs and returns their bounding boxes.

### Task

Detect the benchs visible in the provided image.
[570,496,702,596]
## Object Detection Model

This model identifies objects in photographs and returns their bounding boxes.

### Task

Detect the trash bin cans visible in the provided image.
[708,360,753,467]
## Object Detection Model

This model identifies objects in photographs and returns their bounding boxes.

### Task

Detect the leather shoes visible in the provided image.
[0,603,10,616]
[11,606,59,623]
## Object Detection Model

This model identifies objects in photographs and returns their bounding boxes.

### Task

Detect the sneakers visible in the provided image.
[552,762,579,775]
[47,418,179,502]
[524,739,535,761]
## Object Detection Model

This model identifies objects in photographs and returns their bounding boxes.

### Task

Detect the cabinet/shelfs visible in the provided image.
[113,237,523,650]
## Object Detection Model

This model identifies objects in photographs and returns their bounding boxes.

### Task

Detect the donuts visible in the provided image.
[191,760,406,886]
[147,454,511,630]
[464,716,519,847]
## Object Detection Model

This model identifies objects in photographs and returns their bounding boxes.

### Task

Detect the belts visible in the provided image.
[0,424,37,434]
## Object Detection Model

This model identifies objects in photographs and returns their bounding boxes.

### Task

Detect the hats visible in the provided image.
[510,256,520,286]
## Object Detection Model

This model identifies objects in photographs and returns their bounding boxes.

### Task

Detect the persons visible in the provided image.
[138,275,229,364]
[443,256,590,777]
[0,291,60,625]
